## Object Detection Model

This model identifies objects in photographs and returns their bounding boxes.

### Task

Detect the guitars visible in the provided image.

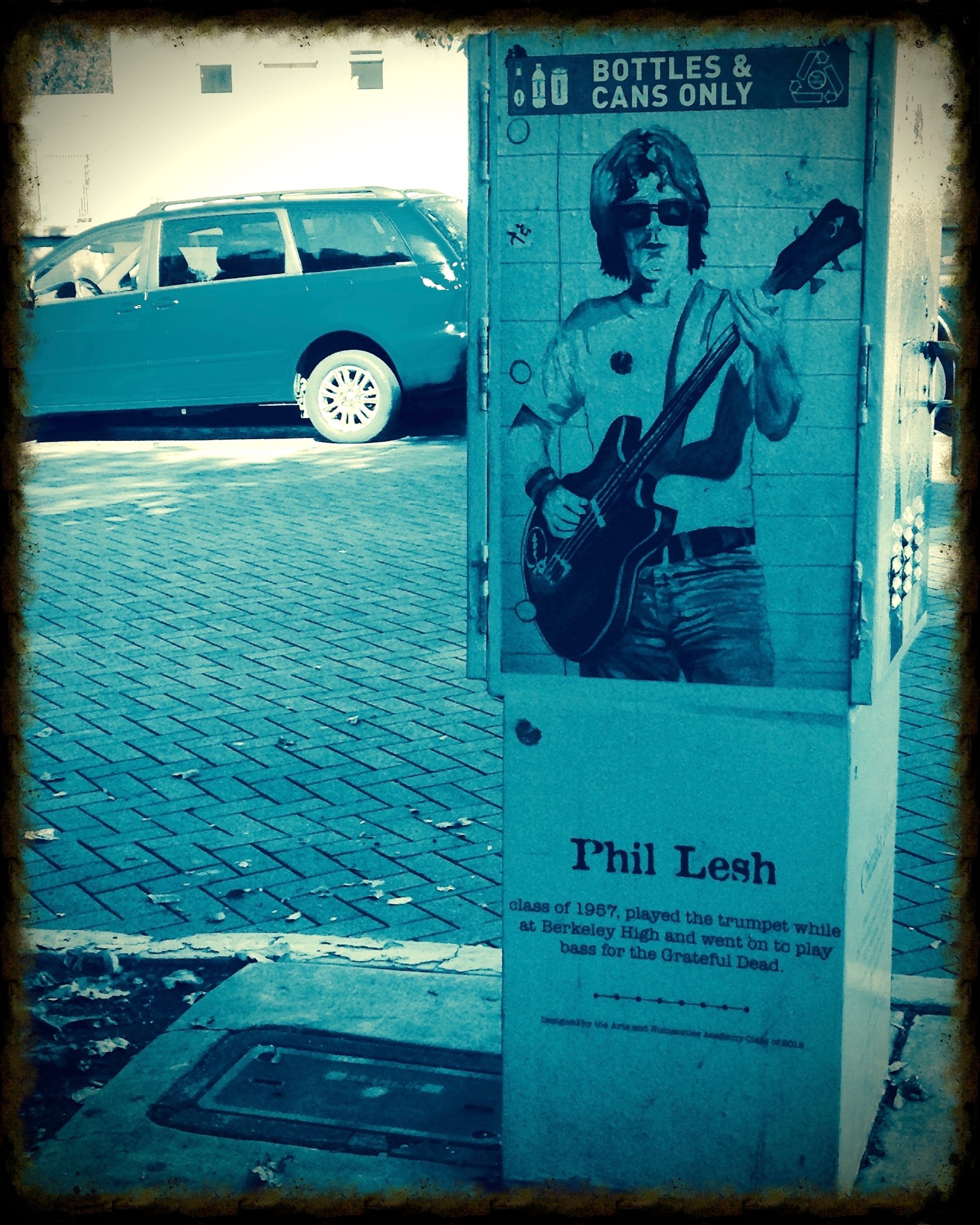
[521,196,866,662]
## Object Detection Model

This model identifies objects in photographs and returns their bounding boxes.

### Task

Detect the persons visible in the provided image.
[508,123,804,686]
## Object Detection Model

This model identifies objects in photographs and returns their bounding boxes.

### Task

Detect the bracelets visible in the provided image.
[524,467,555,498]
[534,477,561,509]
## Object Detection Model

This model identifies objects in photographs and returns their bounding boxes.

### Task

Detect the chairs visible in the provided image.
[158,237,198,287]
[211,240,260,282]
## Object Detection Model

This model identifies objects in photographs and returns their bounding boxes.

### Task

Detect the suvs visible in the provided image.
[16,187,470,445]
